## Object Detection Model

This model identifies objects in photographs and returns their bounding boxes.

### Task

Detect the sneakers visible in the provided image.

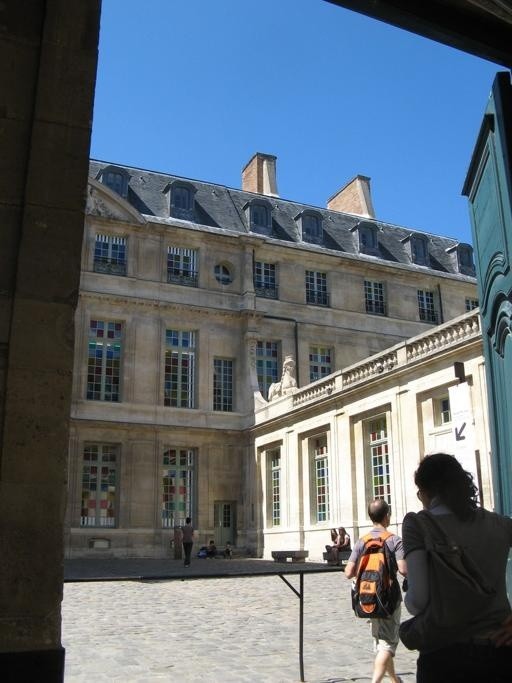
[185,565,190,567]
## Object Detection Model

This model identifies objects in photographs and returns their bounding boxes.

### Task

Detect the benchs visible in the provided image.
[272,551,309,563]
[325,552,352,565]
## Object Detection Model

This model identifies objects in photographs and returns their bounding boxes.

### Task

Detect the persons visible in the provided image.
[331,527,350,566]
[224,541,232,560]
[326,529,338,553]
[343,499,408,683]
[404,454,512,683]
[182,518,194,567]
[206,540,217,560]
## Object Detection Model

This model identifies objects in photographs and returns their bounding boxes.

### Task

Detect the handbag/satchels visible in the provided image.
[400,543,492,650]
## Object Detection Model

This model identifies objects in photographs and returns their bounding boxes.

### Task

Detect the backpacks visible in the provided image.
[352,532,401,619]
